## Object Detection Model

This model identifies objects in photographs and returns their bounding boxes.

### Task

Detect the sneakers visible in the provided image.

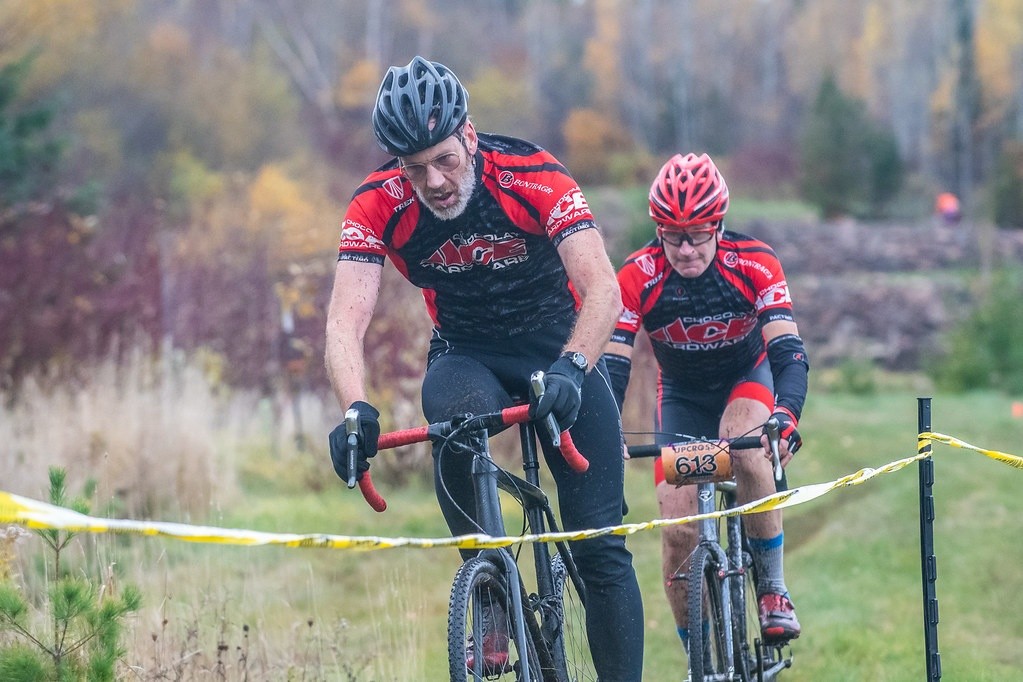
[466,606,511,674]
[756,589,801,638]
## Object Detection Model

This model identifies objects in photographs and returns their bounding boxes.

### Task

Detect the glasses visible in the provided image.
[397,135,466,181]
[656,221,722,248]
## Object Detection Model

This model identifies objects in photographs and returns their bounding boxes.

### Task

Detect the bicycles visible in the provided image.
[345,367,602,682]
[622,416,792,682]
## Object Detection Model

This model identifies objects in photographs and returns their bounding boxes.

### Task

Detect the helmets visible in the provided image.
[648,153,729,228]
[370,55,469,155]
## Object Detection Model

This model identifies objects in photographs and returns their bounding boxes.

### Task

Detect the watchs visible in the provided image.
[557,351,589,379]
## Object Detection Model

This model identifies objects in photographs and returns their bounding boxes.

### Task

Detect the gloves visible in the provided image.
[527,357,585,434]
[328,401,380,489]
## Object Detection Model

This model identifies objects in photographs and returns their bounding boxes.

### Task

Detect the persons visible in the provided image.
[597,152,808,682]
[323,57,644,682]
